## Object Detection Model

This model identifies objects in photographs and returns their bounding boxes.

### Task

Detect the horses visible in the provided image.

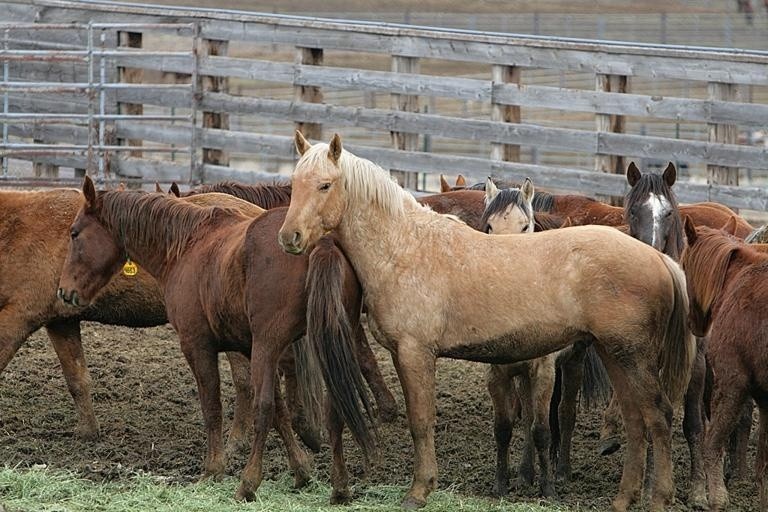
[1,128,768,511]
